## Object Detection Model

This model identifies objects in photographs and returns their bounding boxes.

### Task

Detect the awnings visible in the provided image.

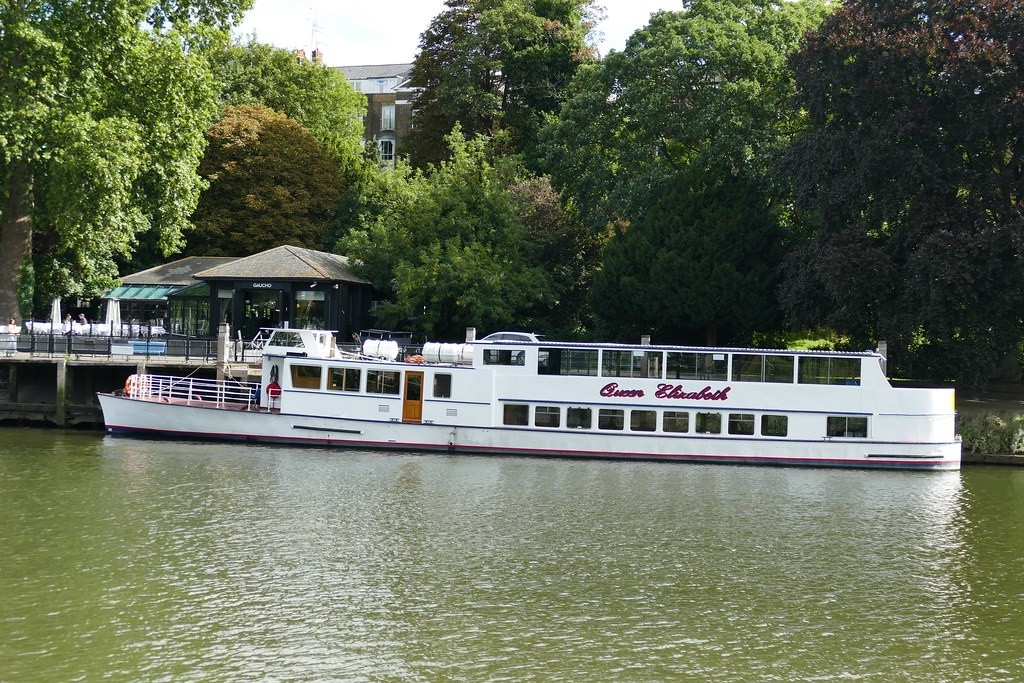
[101,284,185,301]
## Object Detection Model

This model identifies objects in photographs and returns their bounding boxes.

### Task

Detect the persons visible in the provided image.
[5,318,20,357]
[845,372,857,385]
[78,313,87,325]
[63,314,74,325]
[352,332,362,351]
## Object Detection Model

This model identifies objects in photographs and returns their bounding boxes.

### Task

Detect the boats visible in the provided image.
[95,329,962,472]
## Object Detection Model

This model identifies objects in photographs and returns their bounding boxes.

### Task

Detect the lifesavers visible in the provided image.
[125,375,148,394]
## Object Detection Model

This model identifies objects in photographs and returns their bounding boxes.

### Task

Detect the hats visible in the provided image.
[78,314,84,316]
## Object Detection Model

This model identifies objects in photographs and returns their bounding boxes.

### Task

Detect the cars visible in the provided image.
[478,332,550,366]
